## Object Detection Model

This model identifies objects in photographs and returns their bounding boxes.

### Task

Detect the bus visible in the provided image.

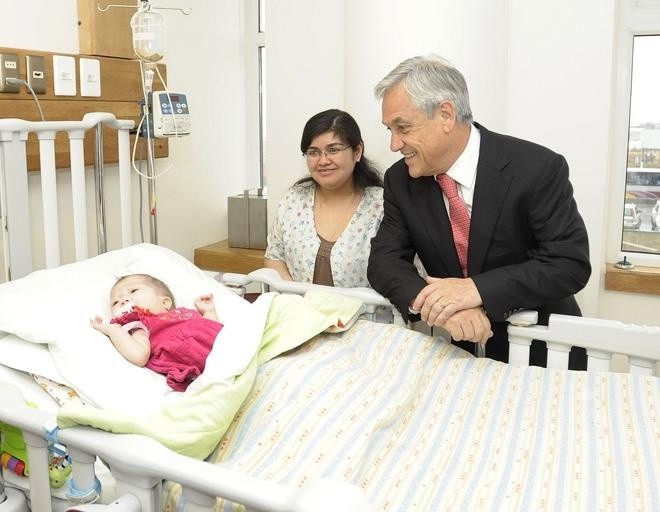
[625,167,660,203]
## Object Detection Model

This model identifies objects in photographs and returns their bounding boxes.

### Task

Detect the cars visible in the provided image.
[624,204,642,229]
[651,200,660,231]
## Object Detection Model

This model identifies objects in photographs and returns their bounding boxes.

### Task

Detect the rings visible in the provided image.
[436,301,446,309]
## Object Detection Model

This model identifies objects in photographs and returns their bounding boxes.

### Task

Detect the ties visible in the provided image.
[438,172,470,277]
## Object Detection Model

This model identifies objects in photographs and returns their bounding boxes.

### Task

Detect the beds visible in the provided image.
[0,110,658,512]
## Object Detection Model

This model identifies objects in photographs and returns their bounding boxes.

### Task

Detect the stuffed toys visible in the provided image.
[1,419,73,489]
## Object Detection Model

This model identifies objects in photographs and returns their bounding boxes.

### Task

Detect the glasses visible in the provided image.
[303,146,350,160]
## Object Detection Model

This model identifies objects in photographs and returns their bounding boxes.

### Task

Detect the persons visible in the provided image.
[368,53,593,369]
[260,108,428,332]
[91,274,226,395]
